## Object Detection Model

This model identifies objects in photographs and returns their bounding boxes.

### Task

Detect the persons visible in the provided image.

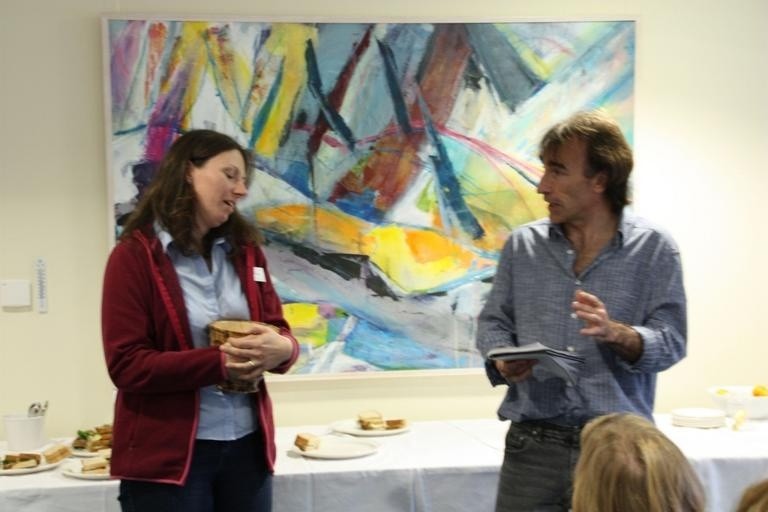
[102,130,299,512]
[736,478,768,512]
[569,411,707,512]
[475,114,687,512]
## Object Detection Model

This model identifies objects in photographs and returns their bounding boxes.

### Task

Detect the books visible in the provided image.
[487,342,587,388]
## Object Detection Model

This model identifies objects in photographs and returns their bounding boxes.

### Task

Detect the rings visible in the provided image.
[249,360,257,368]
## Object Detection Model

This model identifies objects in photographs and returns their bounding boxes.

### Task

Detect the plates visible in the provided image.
[671,407,726,428]
[291,416,412,459]
[0,442,119,479]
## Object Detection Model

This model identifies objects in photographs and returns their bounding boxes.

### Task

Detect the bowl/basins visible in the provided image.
[709,384,768,418]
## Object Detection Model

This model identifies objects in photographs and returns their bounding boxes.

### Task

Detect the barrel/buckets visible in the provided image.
[208,320,279,395]
[4,413,44,449]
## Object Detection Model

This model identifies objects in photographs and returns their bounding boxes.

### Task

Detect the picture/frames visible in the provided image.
[99,16,639,383]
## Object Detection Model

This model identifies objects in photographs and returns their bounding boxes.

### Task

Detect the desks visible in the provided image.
[0,418,768,512]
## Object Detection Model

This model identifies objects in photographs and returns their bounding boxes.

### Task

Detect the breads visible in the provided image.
[3,425,114,475]
[295,433,320,452]
[358,410,405,430]
